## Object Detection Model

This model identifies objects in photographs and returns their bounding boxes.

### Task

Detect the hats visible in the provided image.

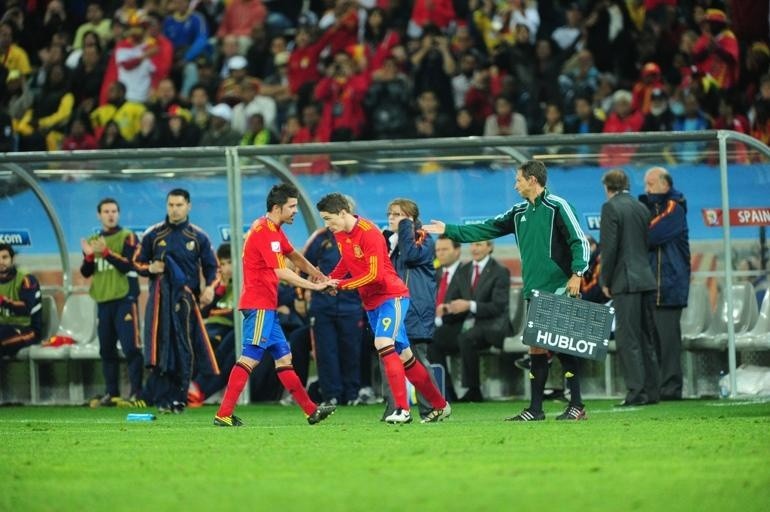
[228,55,250,72]
[642,61,663,79]
[651,89,669,102]
[704,10,729,25]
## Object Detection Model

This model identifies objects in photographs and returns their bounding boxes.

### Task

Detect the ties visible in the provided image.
[437,271,449,306]
[474,265,482,289]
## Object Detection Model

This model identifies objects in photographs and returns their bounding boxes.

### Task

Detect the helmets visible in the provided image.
[128,9,151,30]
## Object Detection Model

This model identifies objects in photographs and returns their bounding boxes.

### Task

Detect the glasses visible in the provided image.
[386,212,404,216]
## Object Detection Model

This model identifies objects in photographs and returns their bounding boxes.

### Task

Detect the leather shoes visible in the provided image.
[459,392,484,403]
[613,399,648,408]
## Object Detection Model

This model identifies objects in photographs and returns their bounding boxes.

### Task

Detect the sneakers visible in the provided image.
[505,408,546,422]
[280,386,386,408]
[541,388,565,401]
[421,401,453,425]
[515,351,554,371]
[556,404,586,421]
[308,405,338,425]
[89,379,205,416]
[386,409,414,423]
[215,415,245,427]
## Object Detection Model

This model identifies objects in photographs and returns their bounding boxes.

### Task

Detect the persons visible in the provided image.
[1,0,769,173]
[1,244,46,359]
[78,161,692,426]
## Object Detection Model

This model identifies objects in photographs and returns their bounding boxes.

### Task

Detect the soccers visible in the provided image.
[358,386,376,404]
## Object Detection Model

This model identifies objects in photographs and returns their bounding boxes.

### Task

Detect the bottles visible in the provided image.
[127,413,156,421]
